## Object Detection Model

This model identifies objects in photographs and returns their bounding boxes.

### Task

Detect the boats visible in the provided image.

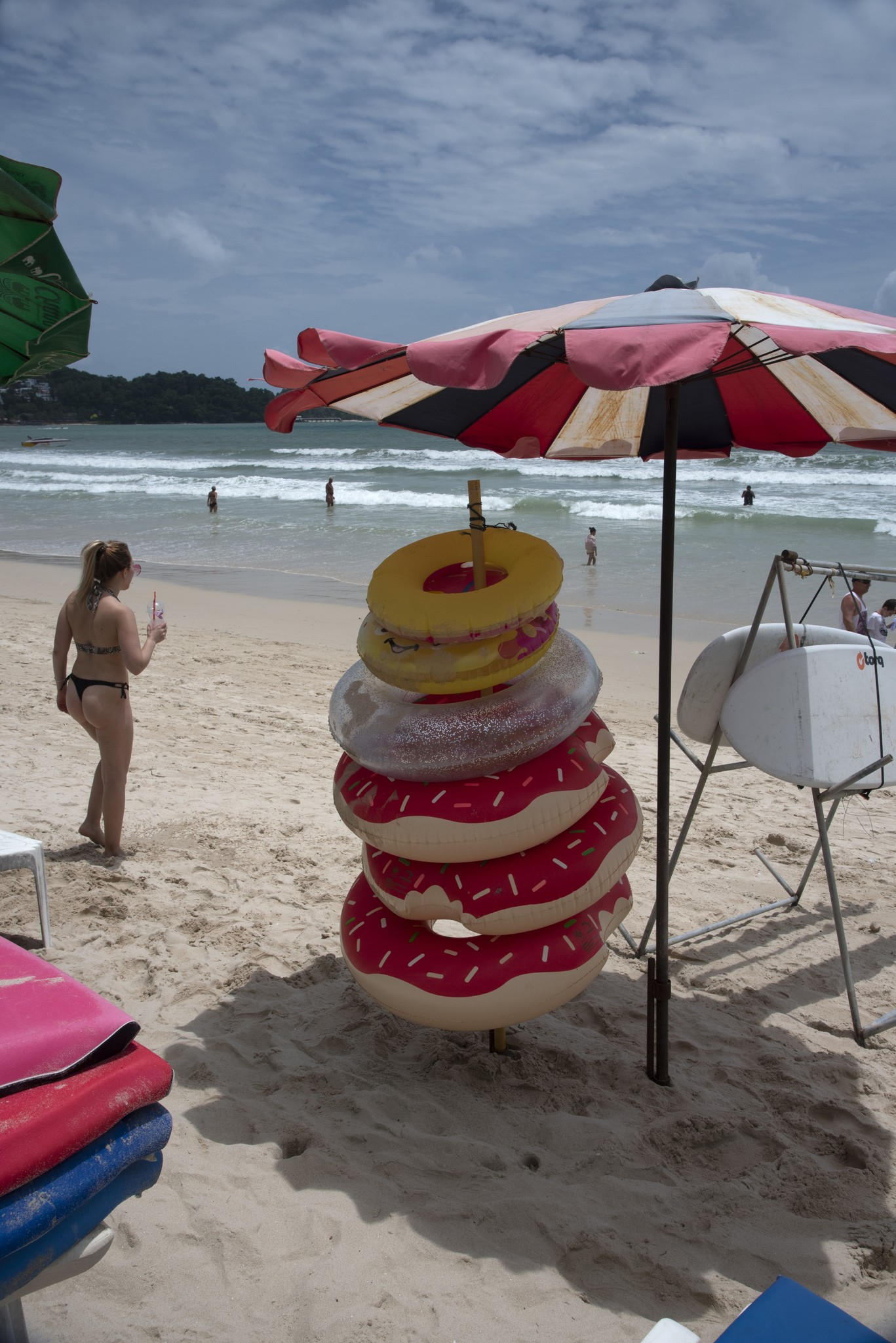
[21,435,71,448]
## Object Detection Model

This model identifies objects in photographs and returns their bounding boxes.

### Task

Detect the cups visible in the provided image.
[145,601,166,630]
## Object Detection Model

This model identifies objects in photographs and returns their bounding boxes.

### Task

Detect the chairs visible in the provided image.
[0,831,52,950]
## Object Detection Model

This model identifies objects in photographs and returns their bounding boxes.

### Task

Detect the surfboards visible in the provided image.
[720,644,896,790]
[677,622,895,746]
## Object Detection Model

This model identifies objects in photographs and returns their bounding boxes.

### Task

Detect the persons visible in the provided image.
[866,599,896,645]
[325,477,335,507]
[207,486,217,513]
[838,571,871,635]
[53,538,167,858]
[585,527,597,566]
[741,485,756,506]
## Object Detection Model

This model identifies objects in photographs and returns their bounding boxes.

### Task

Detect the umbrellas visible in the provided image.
[263,275,896,1091]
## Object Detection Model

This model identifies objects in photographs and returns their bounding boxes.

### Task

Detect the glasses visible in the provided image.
[856,580,871,584]
[126,563,141,576]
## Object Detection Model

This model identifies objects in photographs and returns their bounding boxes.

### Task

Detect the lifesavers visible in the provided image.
[329,628,604,781]
[355,566,563,695]
[367,525,566,644]
[342,863,634,1029]
[332,682,614,864]
[362,760,643,936]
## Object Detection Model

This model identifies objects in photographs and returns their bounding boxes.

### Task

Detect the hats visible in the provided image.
[211,486,216,490]
[852,571,866,582]
